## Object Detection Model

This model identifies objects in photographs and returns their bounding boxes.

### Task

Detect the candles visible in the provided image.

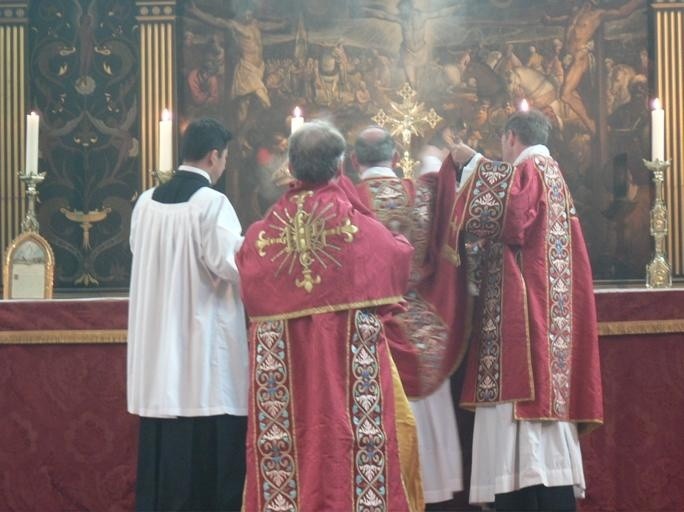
[159,121,173,172]
[25,113,39,175]
[650,110,665,160]
[291,117,303,135]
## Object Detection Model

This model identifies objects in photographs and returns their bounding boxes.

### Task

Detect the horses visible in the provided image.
[508,66,588,142]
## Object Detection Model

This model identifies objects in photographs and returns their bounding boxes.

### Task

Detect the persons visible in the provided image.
[442,111,604,512]
[126,118,250,511]
[346,127,466,512]
[238,123,428,512]
